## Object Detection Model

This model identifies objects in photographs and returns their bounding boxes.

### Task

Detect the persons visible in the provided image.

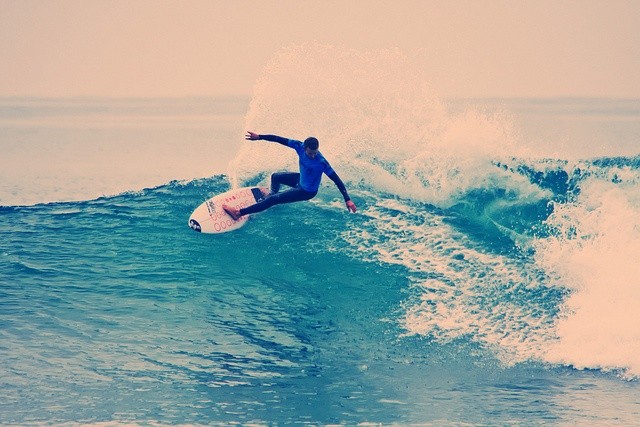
[222,130,357,221]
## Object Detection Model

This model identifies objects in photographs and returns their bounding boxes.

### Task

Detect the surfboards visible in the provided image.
[188,186,267,234]
